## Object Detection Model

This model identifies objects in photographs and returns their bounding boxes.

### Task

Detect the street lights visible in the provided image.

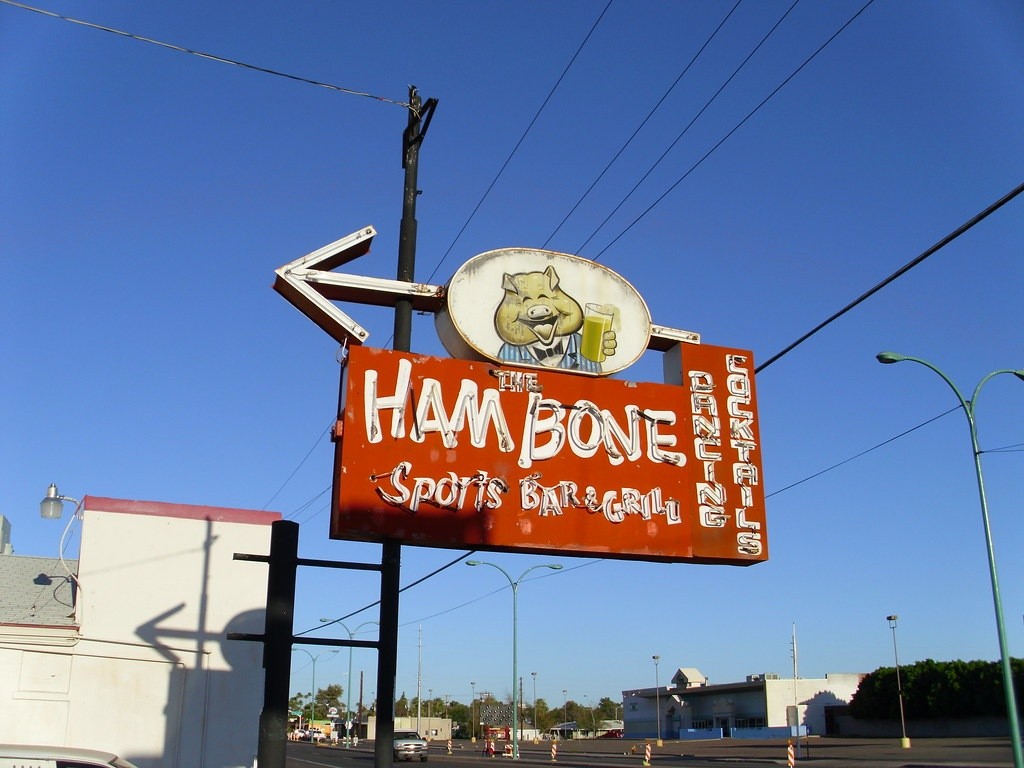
[531,671,539,745]
[887,614,912,749]
[877,350,1024,768]
[653,654,664,748]
[427,690,433,744]
[320,618,378,748]
[291,648,339,743]
[466,560,564,759]
[470,682,477,743]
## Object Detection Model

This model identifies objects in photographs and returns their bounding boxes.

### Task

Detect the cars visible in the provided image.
[304,728,326,743]
[393,730,428,762]
